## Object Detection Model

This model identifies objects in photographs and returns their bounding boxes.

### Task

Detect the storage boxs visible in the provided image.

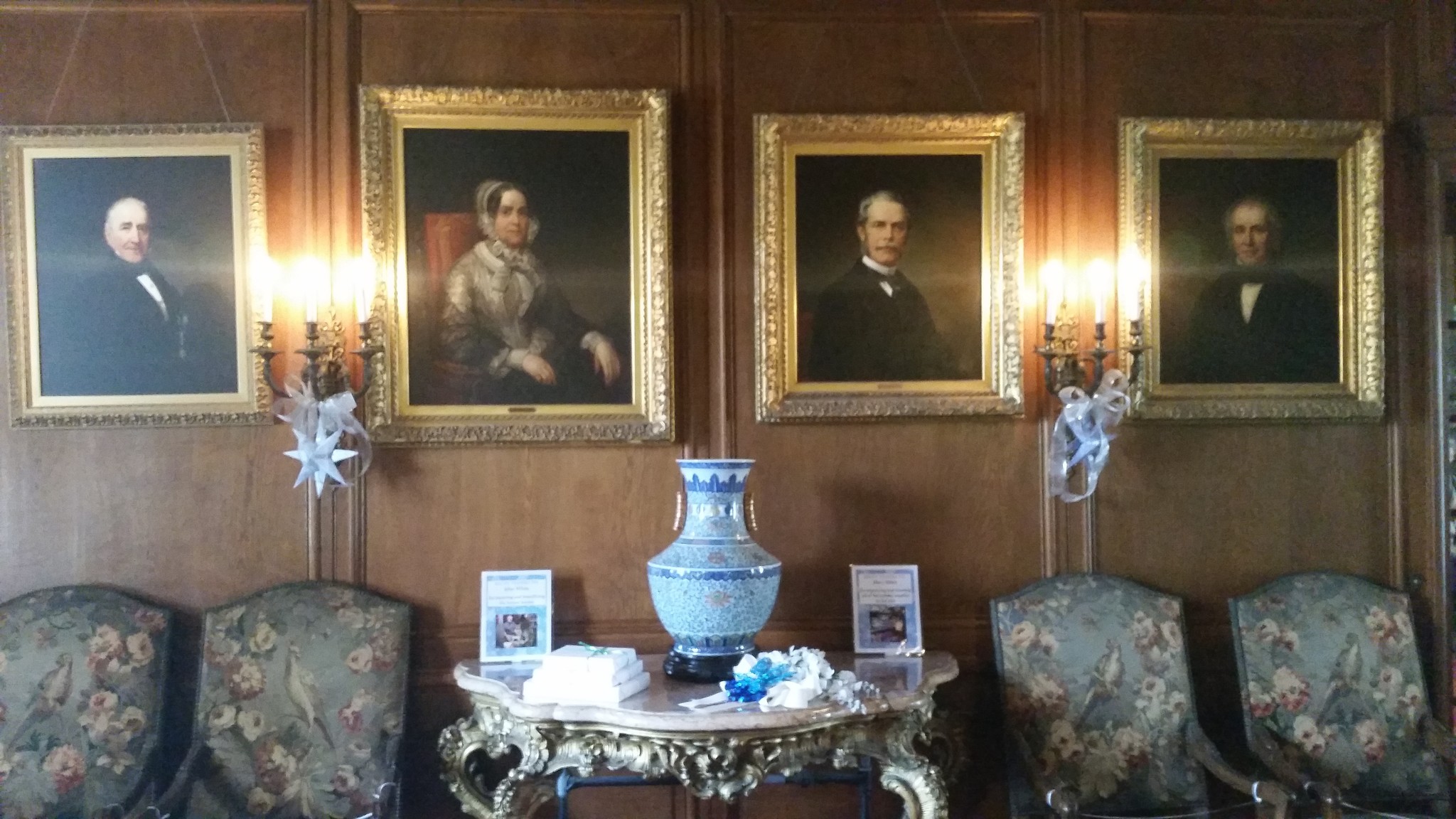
[517,636,650,708]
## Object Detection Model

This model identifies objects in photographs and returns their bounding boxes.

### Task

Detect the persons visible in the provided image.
[892,619,906,641]
[495,614,533,647]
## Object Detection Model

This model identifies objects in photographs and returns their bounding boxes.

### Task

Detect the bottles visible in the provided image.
[645,459,782,682]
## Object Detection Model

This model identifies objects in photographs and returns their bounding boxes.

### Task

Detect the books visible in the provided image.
[523,645,652,704]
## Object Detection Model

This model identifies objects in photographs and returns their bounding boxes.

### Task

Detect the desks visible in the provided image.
[437,651,962,819]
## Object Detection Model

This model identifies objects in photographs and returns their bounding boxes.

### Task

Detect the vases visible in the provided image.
[646,458,784,682]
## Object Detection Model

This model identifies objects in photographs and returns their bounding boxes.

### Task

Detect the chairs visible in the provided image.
[1223,566,1456,819]
[0,586,177,819]
[994,571,1310,819]
[148,577,414,818]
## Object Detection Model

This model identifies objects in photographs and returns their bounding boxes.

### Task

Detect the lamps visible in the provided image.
[245,248,384,433]
[1038,249,1146,409]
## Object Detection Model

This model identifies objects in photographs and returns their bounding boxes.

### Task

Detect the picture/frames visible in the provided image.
[1,120,273,431]
[749,112,1027,419]
[1116,115,1386,426]
[355,82,678,448]
